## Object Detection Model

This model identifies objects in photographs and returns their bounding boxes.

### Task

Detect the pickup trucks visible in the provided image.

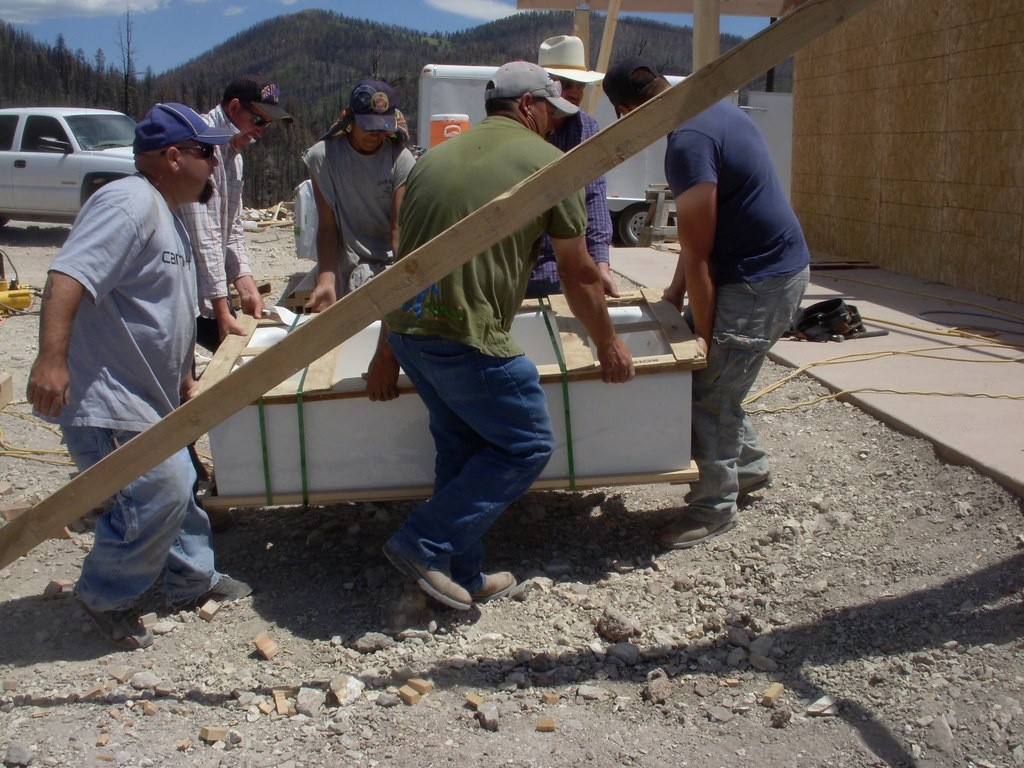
[0,108,139,228]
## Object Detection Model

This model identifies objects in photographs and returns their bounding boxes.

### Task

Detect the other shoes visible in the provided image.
[71,586,153,651]
[202,510,233,531]
[186,574,255,603]
[381,541,516,610]
[654,517,740,549]
[689,472,768,498]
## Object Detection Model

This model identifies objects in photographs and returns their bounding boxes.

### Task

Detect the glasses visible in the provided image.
[251,114,272,128]
[161,142,215,158]
[529,80,562,97]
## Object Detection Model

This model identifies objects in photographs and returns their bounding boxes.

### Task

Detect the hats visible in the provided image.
[224,75,295,125]
[484,60,579,119]
[350,80,398,133]
[537,35,605,83]
[133,102,234,154]
[602,55,659,119]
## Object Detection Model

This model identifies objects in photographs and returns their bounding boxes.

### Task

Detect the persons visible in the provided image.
[176,76,293,531]
[603,56,811,546]
[361,61,634,611]
[25,102,254,649]
[524,34,620,297]
[303,80,417,312]
